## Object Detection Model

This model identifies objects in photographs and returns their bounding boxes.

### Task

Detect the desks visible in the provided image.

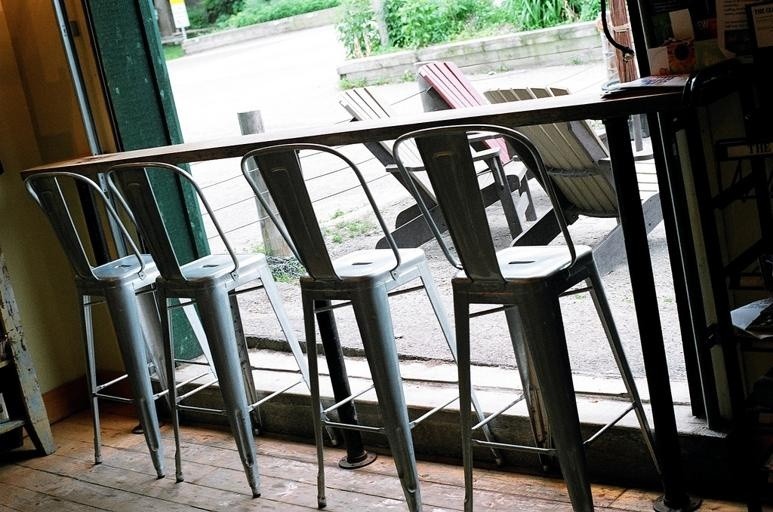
[16,78,707,493]
